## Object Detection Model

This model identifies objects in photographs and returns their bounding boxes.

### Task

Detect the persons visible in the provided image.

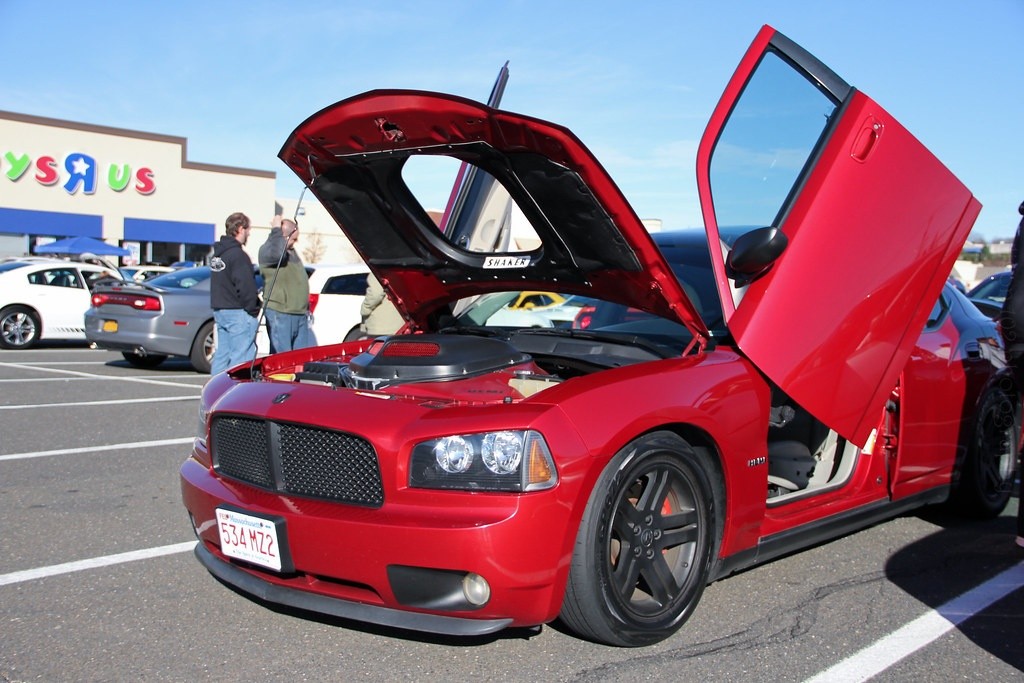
[210,212,262,377]
[360,272,405,339]
[1001,202,1024,552]
[258,215,318,354]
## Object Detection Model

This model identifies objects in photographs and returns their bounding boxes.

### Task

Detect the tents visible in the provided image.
[33,235,132,267]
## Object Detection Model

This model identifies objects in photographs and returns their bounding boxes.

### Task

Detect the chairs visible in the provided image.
[50,274,70,287]
[35,275,47,285]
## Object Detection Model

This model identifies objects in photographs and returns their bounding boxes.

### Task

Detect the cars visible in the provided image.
[214,264,371,367]
[0,257,133,350]
[122,266,173,286]
[84,266,222,375]
[178,24,1020,647]
[964,272,1016,323]
